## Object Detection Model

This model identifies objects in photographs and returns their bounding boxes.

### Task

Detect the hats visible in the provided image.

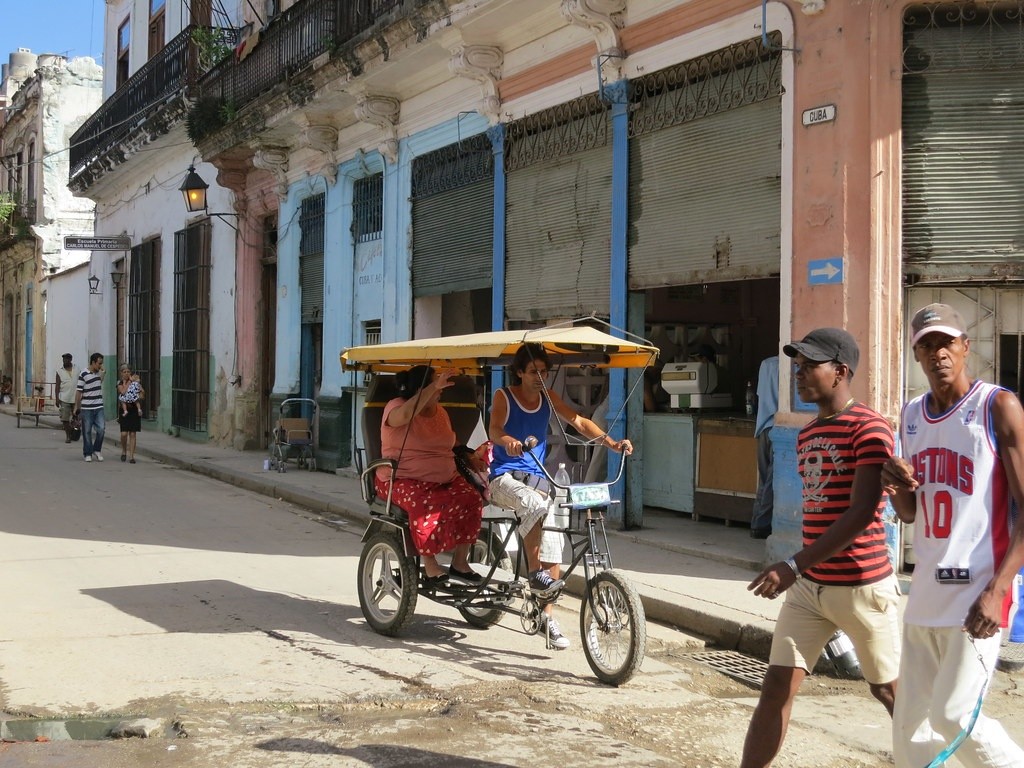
[783,327,860,375]
[912,303,969,347]
[687,344,716,361]
[62,354,72,358]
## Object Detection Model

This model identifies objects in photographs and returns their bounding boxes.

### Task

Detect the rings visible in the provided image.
[128,379,133,383]
[984,630,993,636]
[772,591,780,597]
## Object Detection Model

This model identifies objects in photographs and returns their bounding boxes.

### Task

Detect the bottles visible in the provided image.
[745,379,755,417]
[553,464,570,527]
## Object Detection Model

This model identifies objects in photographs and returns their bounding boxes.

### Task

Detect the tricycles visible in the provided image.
[15,381,59,428]
[353,364,649,687]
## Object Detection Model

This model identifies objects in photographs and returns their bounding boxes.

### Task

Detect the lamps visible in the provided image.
[177,163,238,232]
[88,275,102,294]
[109,270,125,290]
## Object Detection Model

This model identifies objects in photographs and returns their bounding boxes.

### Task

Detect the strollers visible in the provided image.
[267,398,317,473]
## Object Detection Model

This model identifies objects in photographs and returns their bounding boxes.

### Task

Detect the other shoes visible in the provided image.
[130,459,136,463]
[426,573,449,585]
[122,411,128,417]
[84,455,92,462]
[138,409,142,416]
[66,440,71,443]
[93,451,104,461]
[121,455,126,461]
[750,530,772,539]
[449,563,482,581]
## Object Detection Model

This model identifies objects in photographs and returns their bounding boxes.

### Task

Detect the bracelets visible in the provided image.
[784,555,805,583]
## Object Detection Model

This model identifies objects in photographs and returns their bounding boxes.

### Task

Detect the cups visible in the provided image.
[264,460,268,469]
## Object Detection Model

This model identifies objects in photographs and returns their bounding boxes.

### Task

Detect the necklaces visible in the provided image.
[816,394,856,421]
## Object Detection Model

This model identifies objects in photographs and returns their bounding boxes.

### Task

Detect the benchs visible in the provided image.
[360,374,490,518]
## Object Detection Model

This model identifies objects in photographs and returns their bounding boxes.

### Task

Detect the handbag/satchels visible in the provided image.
[69,415,81,441]
[452,444,490,494]
[4,395,11,404]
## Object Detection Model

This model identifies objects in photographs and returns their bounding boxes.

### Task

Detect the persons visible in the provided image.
[750,352,779,539]
[54,352,81,444]
[115,362,146,464]
[582,372,654,483]
[878,302,1023,768]
[74,352,107,462]
[484,342,633,649]
[374,365,485,584]
[741,325,904,767]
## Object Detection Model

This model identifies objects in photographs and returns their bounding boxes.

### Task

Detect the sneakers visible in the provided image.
[528,568,565,595]
[532,612,570,648]
